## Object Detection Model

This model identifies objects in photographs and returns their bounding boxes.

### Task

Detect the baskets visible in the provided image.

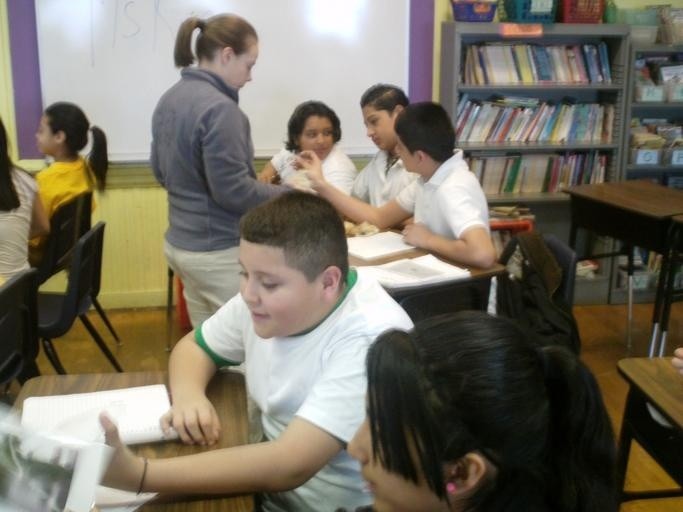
[451,0,498,22]
[560,0,605,24]
[504,0,556,24]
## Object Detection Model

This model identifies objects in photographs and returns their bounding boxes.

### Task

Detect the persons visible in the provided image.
[28,100,109,267]
[97,188,418,511]
[259,100,360,198]
[351,83,421,230]
[0,117,51,288]
[348,308,622,512]
[148,11,288,333]
[670,346,683,378]
[288,100,496,270]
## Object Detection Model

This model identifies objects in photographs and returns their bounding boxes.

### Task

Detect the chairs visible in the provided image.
[0,187,122,385]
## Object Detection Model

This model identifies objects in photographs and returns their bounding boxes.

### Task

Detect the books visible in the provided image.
[461,40,615,86]
[457,92,615,145]
[463,149,606,195]
[20,383,179,448]
[347,231,417,261]
[374,252,473,289]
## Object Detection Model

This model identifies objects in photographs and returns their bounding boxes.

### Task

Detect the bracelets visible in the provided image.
[136,457,148,496]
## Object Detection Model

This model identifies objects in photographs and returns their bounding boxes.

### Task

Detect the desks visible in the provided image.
[561,180,683,358]
[347,225,507,323]
[14,370,254,512]
[615,358,683,512]
